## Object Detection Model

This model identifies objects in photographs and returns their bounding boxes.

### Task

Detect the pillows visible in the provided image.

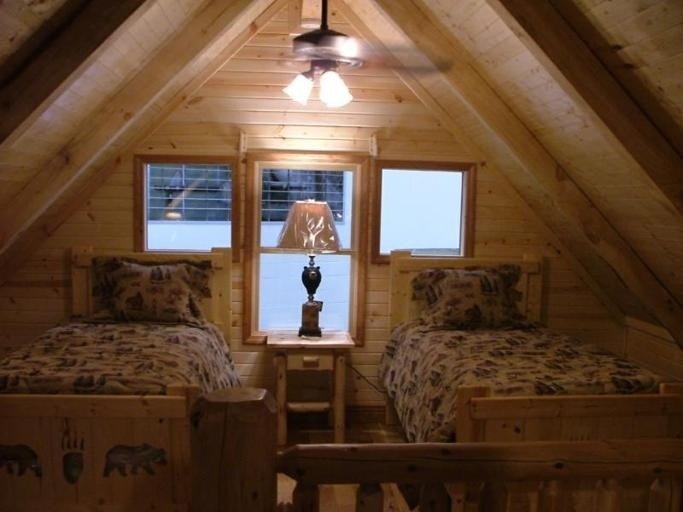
[88,255,214,330]
[410,262,525,330]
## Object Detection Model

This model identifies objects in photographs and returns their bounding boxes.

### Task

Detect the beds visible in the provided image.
[0,243,236,510]
[382,247,683,444]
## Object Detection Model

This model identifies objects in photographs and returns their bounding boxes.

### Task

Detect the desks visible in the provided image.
[264,332,357,443]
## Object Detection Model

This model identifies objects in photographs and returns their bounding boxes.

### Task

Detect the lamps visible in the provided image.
[281,1,366,112]
[277,198,343,337]
